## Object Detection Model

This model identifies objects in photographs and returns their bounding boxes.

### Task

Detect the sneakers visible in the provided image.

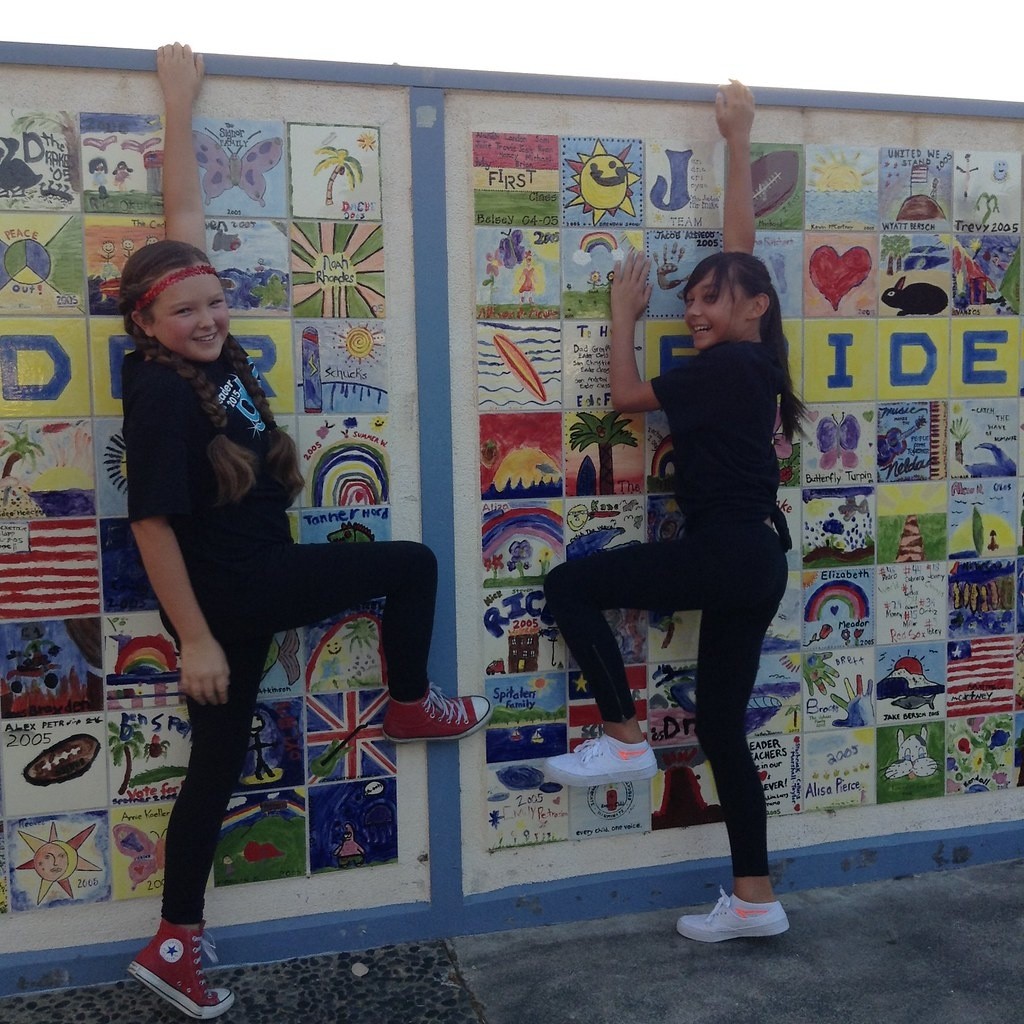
[677,885,789,943]
[383,682,493,744]
[127,918,234,1020]
[547,734,658,786]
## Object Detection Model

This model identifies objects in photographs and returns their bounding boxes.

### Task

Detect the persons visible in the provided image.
[539,78,806,943]
[119,42,494,1019]
